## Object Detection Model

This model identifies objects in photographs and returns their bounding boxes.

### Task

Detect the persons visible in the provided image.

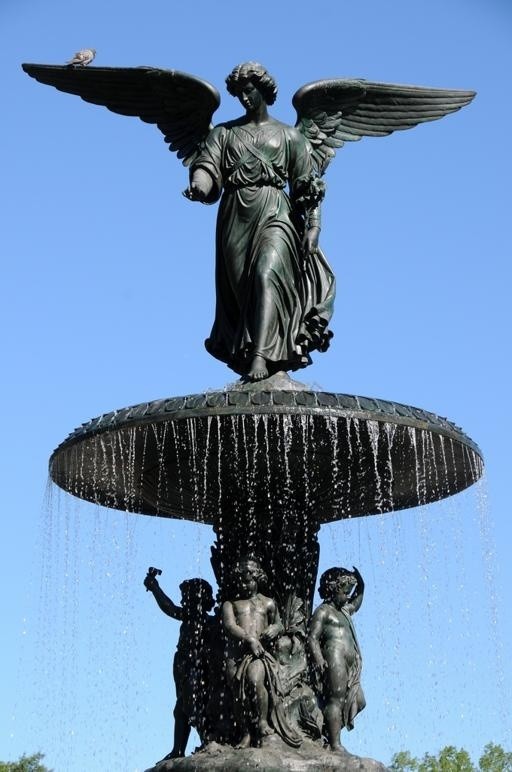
[177,58,334,383]
[142,571,218,762]
[220,554,283,751]
[308,562,369,752]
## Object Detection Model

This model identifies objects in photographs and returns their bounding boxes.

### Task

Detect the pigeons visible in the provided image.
[65,48,97,67]
